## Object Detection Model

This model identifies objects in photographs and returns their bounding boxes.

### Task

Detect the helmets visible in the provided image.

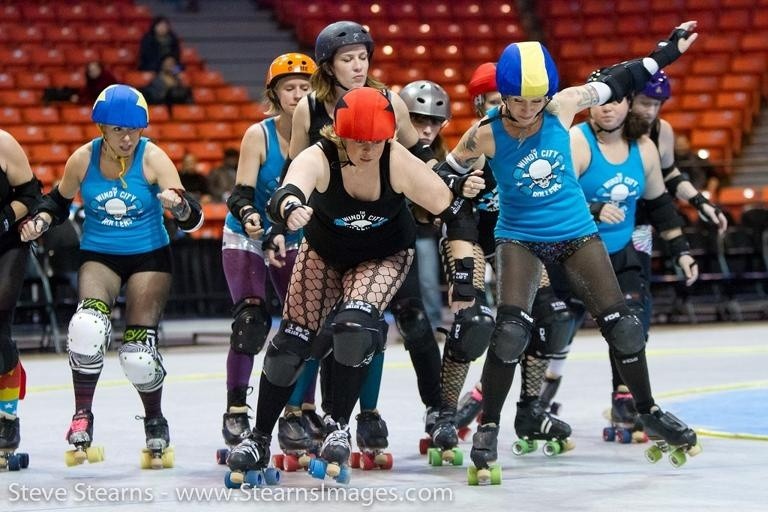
[400,80,450,121]
[266,54,319,89]
[495,41,559,99]
[588,65,671,102]
[469,61,497,96]
[314,20,373,66]
[333,86,395,142]
[92,85,148,128]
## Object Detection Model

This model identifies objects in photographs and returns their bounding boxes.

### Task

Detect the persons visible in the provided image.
[138,14,181,71]
[178,17,733,453]
[70,61,117,105]
[0,129,43,452]
[227,86,480,472]
[143,53,193,104]
[18,84,205,451]
[432,21,700,465]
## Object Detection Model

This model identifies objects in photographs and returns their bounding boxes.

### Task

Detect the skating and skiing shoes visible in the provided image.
[66,412,104,467]
[603,391,647,442]
[417,388,503,485]
[514,400,574,456]
[140,414,174,470]
[539,371,562,416]
[1,414,29,472]
[217,403,393,488]
[640,405,701,467]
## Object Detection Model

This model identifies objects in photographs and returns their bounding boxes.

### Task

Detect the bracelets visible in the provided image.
[33,214,50,233]
[168,188,189,219]
